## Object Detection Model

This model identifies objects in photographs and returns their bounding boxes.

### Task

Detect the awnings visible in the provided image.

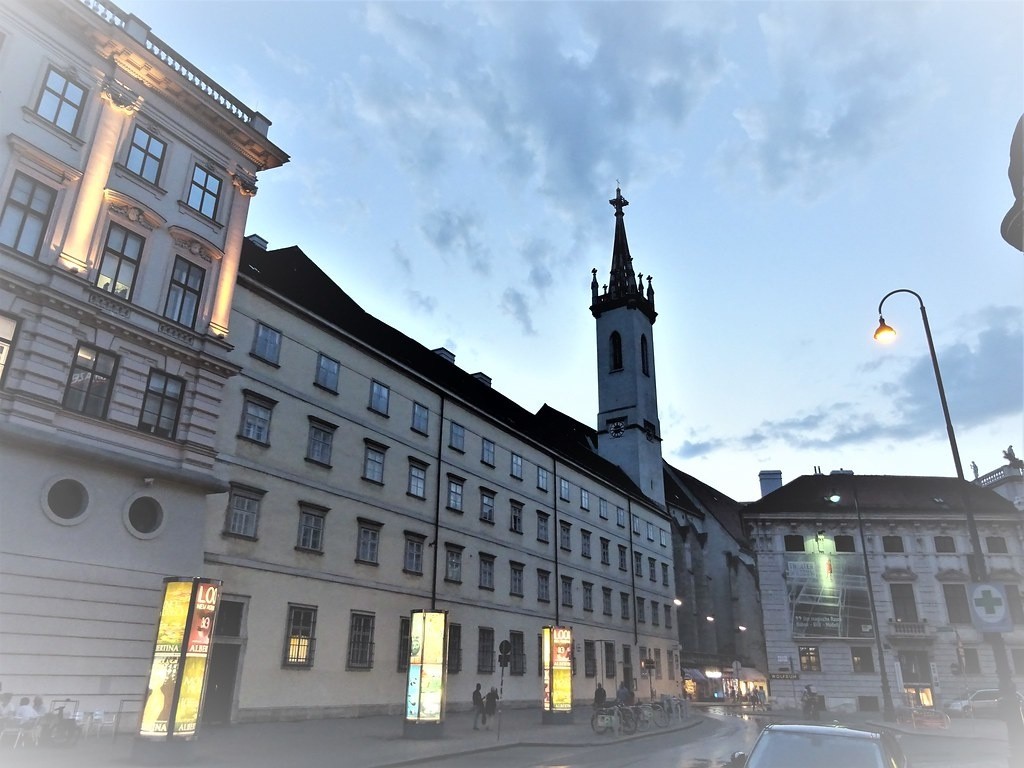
[683,667,706,682]
[731,668,767,682]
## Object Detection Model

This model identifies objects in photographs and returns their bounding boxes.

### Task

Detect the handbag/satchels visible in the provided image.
[481,713,486,725]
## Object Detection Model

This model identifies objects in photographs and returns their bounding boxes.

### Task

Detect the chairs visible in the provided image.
[0,714,118,750]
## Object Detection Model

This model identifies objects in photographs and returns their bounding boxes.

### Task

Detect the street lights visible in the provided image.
[825,473,894,722]
[872,287,1023,768]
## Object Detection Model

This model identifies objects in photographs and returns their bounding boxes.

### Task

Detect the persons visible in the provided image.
[726,685,766,712]
[617,682,629,705]
[595,683,606,713]
[486,687,499,730]
[0,693,48,744]
[473,683,485,729]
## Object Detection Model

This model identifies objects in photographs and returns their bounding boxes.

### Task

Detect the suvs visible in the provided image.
[945,686,1000,716]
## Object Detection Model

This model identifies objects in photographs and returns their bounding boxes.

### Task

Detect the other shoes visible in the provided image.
[473,728,479,730]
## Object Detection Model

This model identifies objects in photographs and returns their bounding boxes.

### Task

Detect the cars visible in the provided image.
[730,721,907,768]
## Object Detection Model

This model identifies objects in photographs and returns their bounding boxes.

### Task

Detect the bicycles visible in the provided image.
[586,699,671,736]
